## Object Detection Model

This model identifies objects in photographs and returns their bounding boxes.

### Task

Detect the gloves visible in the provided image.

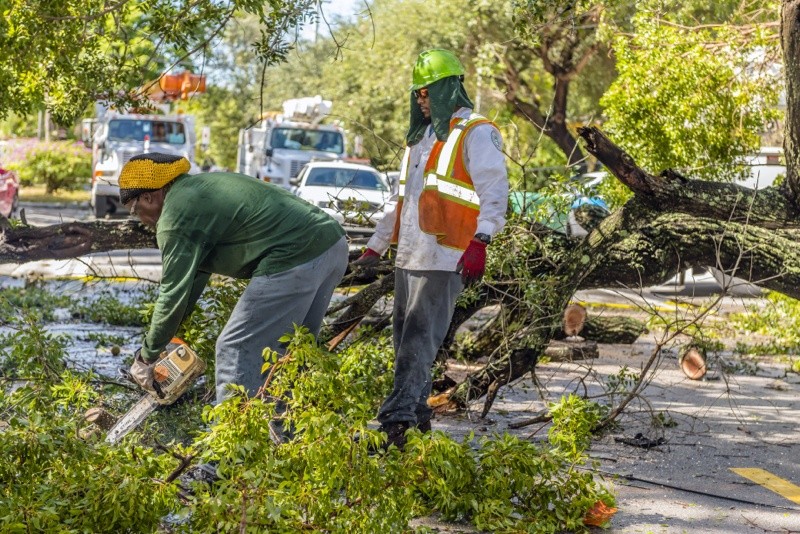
[351,248,381,278]
[456,239,487,288]
[129,347,159,399]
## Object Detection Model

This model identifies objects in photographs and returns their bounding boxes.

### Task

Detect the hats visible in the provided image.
[118,152,191,205]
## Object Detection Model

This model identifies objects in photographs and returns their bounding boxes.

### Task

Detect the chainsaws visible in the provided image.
[105,338,205,445]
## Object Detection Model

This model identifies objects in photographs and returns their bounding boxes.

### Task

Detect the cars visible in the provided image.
[509,164,611,238]
[288,160,391,238]
[0,167,21,220]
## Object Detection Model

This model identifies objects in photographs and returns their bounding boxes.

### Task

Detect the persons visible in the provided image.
[117,153,349,501]
[335,48,509,456]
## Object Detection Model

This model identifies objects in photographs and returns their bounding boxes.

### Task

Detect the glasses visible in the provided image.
[129,195,140,217]
[413,88,429,99]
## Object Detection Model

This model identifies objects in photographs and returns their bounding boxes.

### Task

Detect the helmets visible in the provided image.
[412,48,465,91]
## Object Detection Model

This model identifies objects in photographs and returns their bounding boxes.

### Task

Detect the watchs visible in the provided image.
[474,232,495,245]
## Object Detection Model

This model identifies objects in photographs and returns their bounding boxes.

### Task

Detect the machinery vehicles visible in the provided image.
[78,101,227,219]
[234,93,349,190]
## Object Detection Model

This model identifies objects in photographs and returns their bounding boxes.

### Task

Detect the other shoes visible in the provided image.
[353,420,432,458]
